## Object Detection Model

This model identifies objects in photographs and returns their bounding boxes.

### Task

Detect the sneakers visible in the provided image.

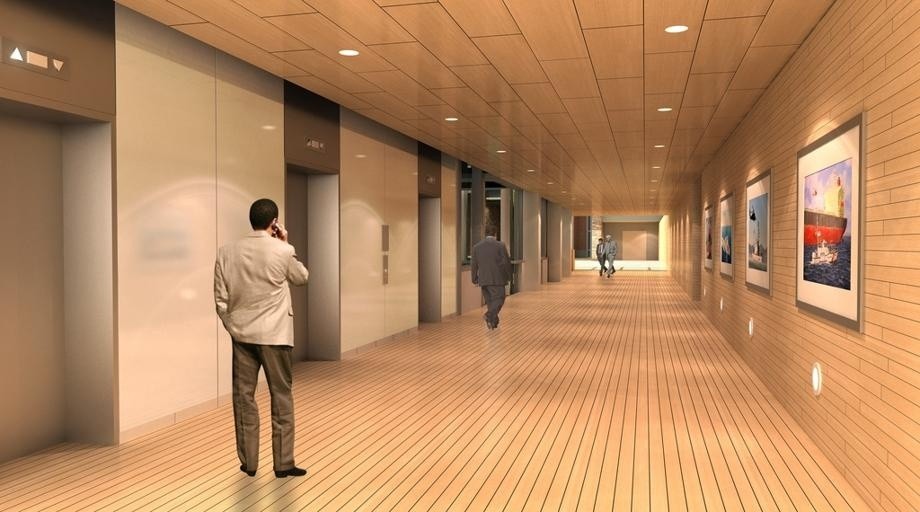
[600,268,616,278]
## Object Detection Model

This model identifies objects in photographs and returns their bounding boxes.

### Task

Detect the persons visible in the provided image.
[600,235,617,278]
[212,198,310,478]
[470,225,513,329]
[596,238,608,276]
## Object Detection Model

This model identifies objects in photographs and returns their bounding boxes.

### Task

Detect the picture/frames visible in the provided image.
[796,110,866,335]
[704,201,715,271]
[719,188,735,280]
[745,167,772,296]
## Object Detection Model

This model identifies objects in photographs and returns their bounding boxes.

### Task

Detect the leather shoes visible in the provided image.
[485,313,499,331]
[274,465,307,478]
[240,464,255,477]
